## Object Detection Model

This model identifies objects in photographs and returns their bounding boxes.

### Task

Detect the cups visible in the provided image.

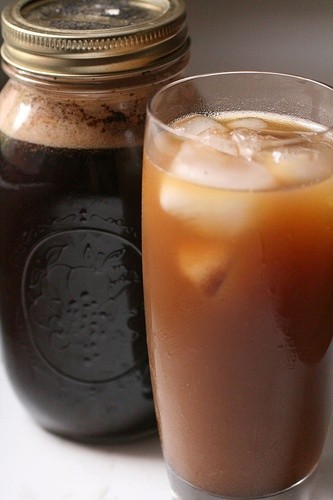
[144,68,333,500]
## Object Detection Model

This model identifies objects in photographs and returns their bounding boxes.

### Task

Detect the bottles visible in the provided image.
[0,0,207,443]
